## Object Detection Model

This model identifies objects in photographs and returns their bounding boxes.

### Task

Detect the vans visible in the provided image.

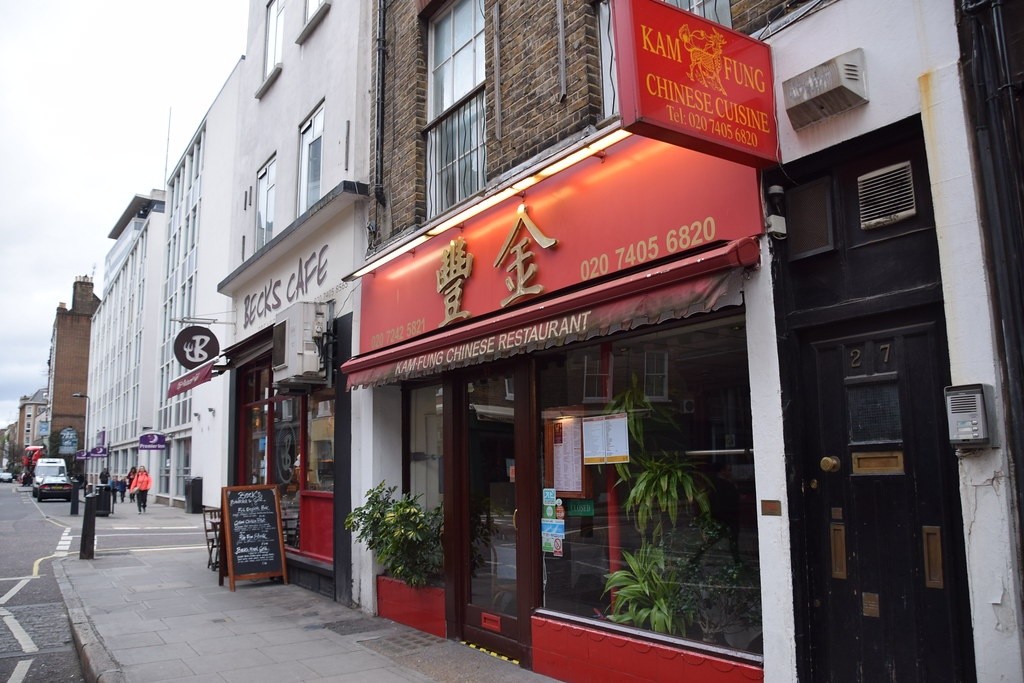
[33,458,67,497]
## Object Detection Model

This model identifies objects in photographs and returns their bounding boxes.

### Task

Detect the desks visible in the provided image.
[209,517,222,572]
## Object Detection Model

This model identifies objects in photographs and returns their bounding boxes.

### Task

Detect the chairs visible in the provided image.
[202,508,221,570]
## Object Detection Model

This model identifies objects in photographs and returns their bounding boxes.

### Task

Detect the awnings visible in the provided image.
[169,322,278,399]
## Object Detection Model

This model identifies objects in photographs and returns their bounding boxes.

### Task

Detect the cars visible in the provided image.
[0,473,13,483]
[37,476,72,502]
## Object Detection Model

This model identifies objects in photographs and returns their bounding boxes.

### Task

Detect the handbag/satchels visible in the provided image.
[133,487,139,494]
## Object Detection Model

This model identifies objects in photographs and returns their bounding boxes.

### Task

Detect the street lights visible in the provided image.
[72,392,90,497]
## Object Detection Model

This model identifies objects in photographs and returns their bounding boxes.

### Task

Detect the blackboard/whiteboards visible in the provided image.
[222,485,289,592]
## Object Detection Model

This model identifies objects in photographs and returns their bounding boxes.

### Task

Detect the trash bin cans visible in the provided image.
[94,485,112,516]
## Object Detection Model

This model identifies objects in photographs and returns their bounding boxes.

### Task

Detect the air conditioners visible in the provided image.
[680,399,696,414]
[270,300,327,394]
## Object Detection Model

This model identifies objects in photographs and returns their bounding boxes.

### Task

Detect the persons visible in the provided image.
[119,474,127,502]
[100,468,110,484]
[127,467,136,503]
[130,465,151,514]
[110,475,119,503]
